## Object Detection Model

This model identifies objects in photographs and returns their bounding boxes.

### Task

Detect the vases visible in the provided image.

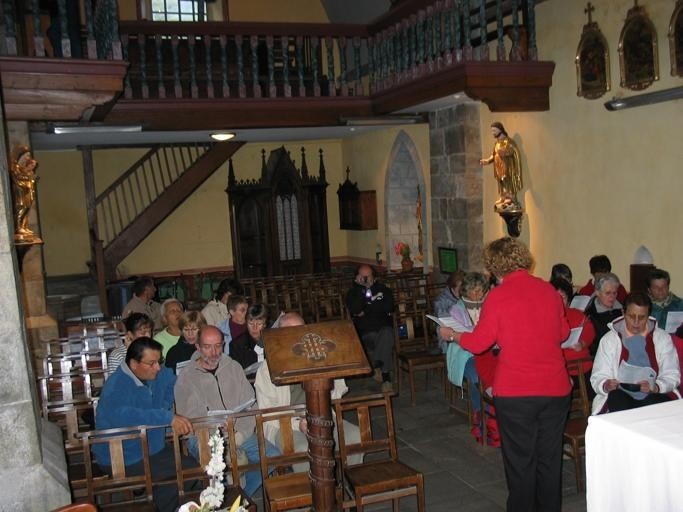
[401,256,413,272]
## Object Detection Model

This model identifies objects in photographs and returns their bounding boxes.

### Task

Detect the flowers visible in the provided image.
[394,241,410,258]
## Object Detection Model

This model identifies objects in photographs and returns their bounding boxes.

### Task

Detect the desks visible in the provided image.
[584,395,682,512]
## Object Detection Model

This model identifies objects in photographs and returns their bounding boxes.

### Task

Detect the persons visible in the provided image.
[11,144,40,234]
[346,265,395,397]
[479,122,522,210]
[25,160,37,234]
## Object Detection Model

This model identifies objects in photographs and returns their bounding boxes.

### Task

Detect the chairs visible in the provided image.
[36,263,346,511]
[341,264,598,447]
[561,358,593,510]
[331,393,426,511]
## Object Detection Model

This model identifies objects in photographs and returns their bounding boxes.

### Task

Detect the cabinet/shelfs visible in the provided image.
[333,163,378,231]
[220,142,331,281]
